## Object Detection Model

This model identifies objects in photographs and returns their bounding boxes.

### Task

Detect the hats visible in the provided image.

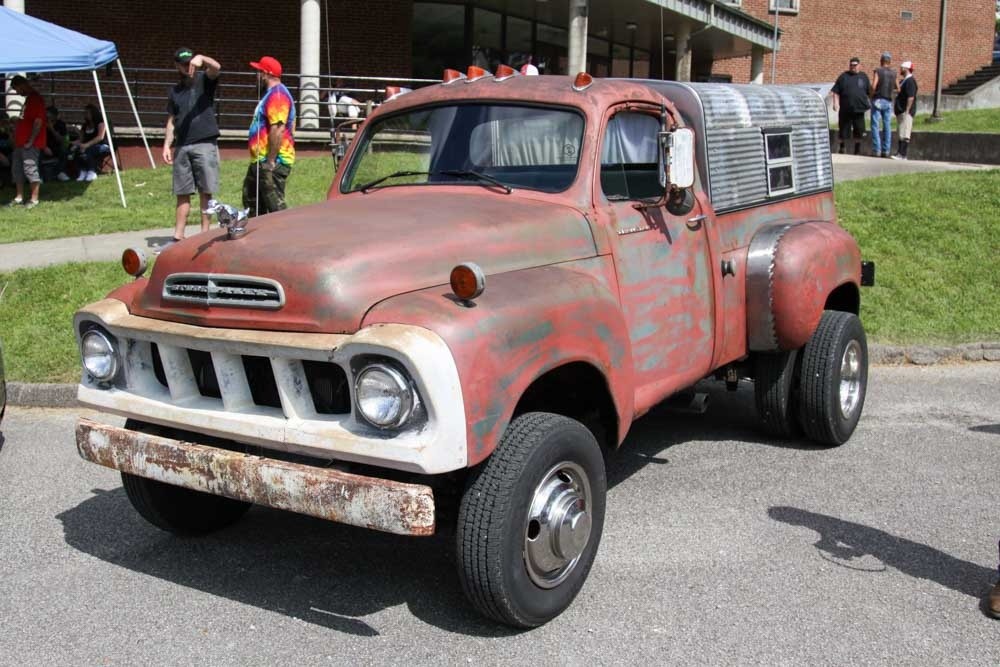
[900,61,914,69]
[175,47,193,65]
[881,52,892,59]
[249,56,282,77]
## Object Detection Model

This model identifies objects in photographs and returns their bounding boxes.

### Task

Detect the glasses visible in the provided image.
[850,64,859,66]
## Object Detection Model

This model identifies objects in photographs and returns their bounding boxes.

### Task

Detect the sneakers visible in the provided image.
[77,170,87,181]
[5,199,23,208]
[893,154,906,160]
[24,199,39,210]
[57,172,70,181]
[151,237,181,254]
[86,171,97,180]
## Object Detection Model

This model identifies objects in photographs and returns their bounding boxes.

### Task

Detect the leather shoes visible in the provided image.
[986,581,1000,620]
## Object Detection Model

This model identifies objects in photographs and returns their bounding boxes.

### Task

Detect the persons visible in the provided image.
[8,75,47,208]
[831,58,871,155]
[870,54,901,157]
[44,106,71,181]
[163,48,221,244]
[0,111,14,184]
[891,62,917,160]
[242,56,297,218]
[321,73,359,129]
[73,104,111,181]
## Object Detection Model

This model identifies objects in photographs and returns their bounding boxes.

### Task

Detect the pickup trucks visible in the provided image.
[72,64,875,631]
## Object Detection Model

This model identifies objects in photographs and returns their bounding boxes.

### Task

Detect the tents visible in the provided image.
[0,6,155,208]
